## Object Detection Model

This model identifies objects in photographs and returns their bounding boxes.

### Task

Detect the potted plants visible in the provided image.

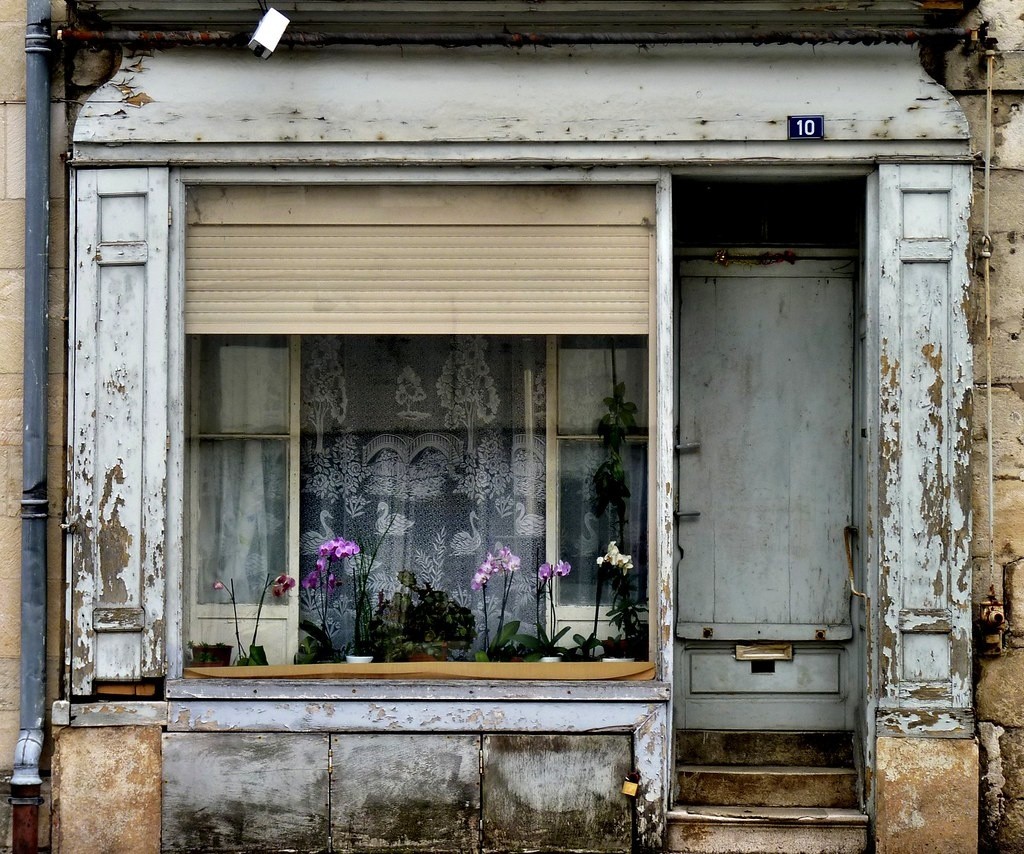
[190,642,234,665]
[381,571,475,662]
[298,637,319,665]
[345,562,374,663]
[188,652,213,667]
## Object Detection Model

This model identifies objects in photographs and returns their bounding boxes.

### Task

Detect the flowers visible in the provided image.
[537,560,573,653]
[212,574,296,666]
[597,541,635,639]
[301,537,359,632]
[467,547,521,659]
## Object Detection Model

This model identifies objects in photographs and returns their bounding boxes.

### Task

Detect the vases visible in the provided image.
[538,656,563,663]
[603,641,637,657]
